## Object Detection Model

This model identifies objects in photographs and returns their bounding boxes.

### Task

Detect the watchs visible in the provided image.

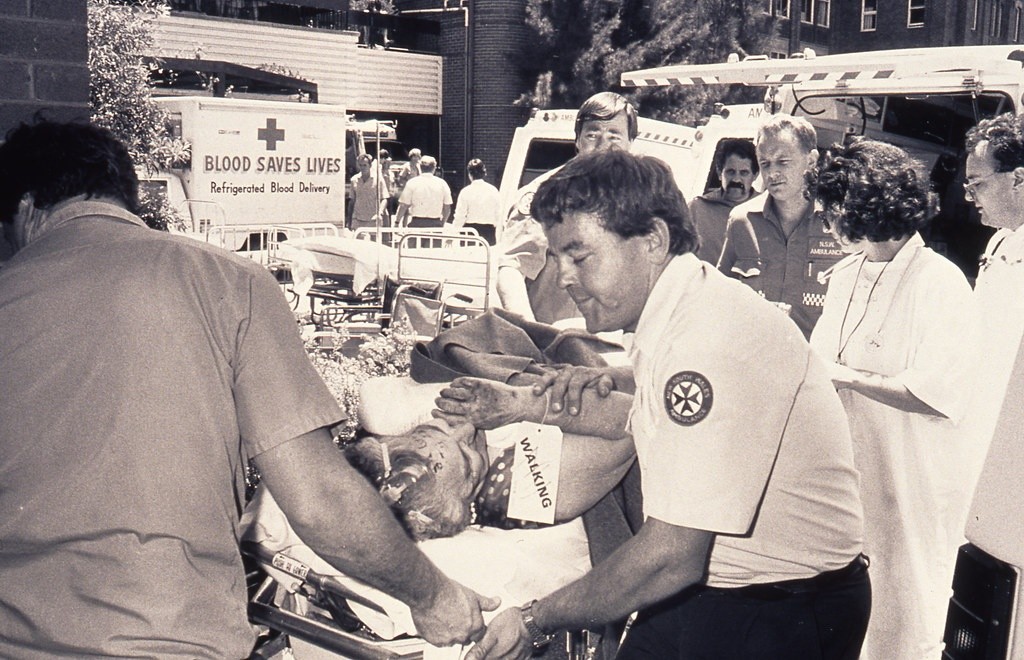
[520,598,562,647]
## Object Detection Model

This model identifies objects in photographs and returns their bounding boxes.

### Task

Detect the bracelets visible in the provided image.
[395,221,399,224]
[533,391,548,426]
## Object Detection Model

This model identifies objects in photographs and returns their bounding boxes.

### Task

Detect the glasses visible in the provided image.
[962,170,1000,195]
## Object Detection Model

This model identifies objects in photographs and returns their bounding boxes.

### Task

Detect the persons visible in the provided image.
[0,106,504,659]
[683,139,761,269]
[454,158,504,248]
[712,113,854,341]
[343,372,636,544]
[469,132,873,660]
[497,92,640,335]
[393,155,453,227]
[807,134,992,660]
[951,113,1024,426]
[348,155,391,231]
[373,148,424,200]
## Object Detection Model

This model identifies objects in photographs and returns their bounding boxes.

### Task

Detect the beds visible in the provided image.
[239,517,602,660]
[207,222,500,326]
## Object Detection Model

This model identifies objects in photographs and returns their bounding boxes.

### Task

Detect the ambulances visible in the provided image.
[498,106,693,215]
[121,95,347,258]
[686,103,768,213]
[347,116,411,198]
[619,42,1019,290]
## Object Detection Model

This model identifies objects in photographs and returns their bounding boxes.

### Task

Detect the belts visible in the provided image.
[696,561,868,598]
